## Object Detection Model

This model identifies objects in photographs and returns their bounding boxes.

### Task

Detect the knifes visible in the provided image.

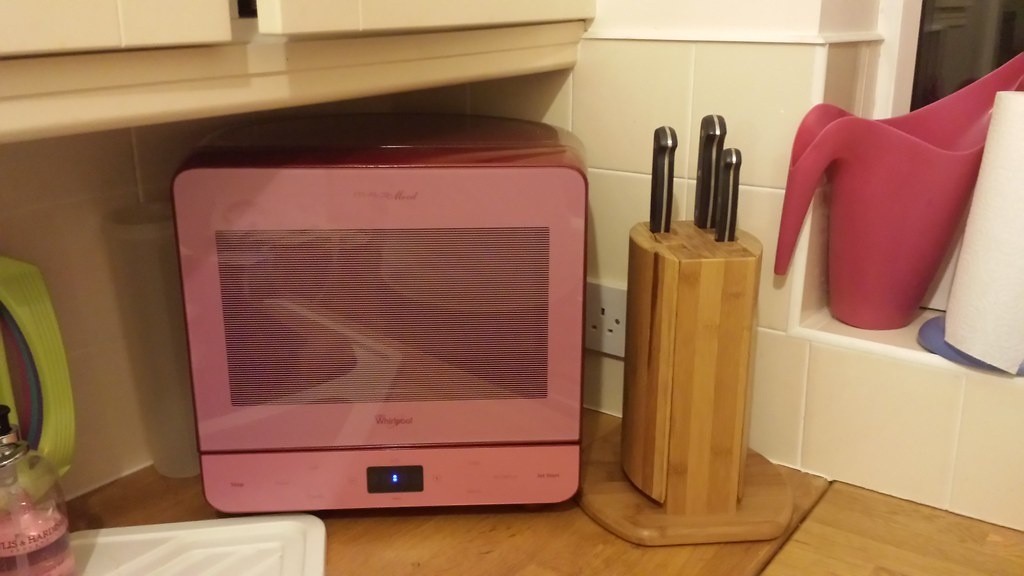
[648,114,745,244]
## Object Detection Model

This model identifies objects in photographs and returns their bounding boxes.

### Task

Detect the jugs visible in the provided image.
[773,51,1024,329]
